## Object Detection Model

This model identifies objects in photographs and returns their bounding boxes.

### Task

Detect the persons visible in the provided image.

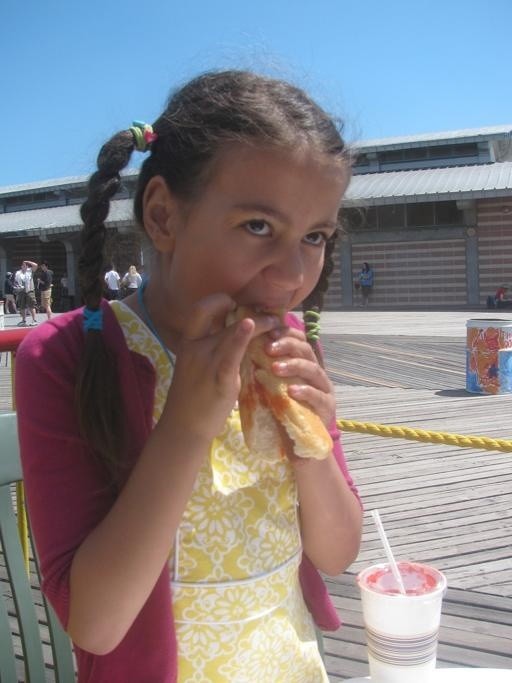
[104,263,143,301]
[358,261,373,308]
[14,70,363,683]
[3,260,67,327]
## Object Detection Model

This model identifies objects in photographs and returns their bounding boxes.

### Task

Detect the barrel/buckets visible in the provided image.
[465,317,512,396]
[355,508,446,681]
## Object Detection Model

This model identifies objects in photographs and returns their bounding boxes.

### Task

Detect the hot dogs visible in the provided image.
[227,304,334,462]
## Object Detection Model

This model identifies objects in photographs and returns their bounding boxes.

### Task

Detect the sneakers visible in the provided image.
[29,320,40,326]
[17,321,28,326]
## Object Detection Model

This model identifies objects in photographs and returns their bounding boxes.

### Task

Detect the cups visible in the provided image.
[355,563,449,678]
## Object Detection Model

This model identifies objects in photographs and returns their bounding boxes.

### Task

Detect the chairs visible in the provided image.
[0,412,325,682]
